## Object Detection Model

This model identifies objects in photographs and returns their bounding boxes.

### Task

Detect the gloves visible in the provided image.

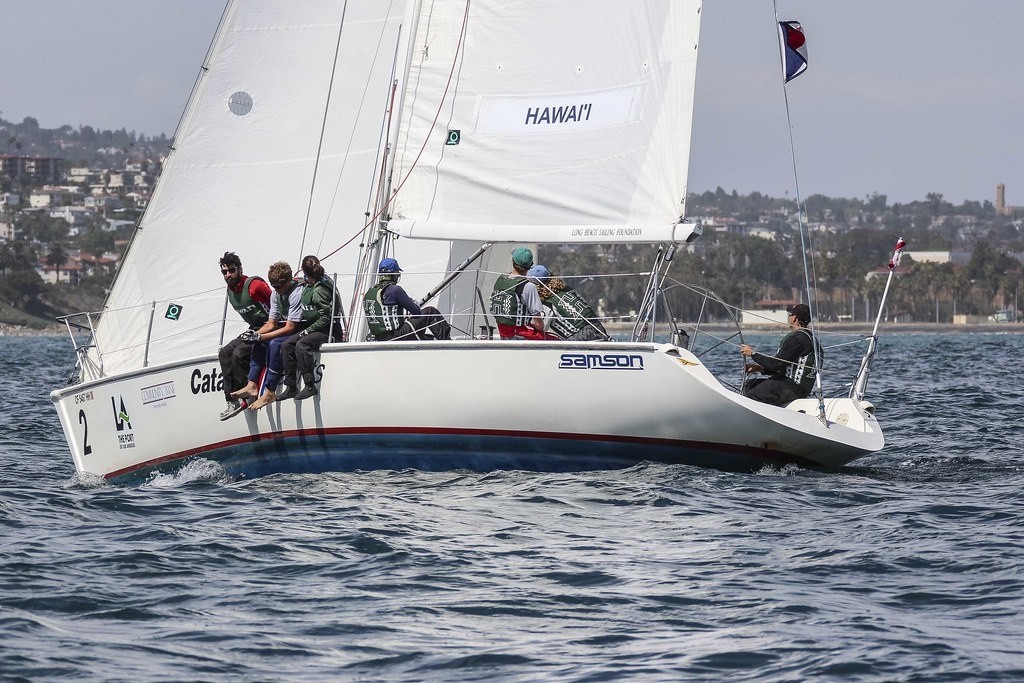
[240,329,261,343]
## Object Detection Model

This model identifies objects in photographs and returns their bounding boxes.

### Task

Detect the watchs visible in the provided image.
[751,351,756,357]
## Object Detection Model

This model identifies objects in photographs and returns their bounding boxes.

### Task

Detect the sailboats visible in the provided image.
[48,0,905,489]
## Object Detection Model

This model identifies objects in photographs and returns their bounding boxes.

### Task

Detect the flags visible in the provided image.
[888,239,906,271]
[779,19,808,84]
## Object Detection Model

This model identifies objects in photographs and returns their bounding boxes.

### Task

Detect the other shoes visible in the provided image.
[275,389,299,400]
[295,383,318,399]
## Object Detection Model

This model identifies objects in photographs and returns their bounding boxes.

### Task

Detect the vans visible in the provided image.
[997,310,1012,322]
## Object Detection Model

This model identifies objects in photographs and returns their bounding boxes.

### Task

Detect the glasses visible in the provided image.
[272,282,286,289]
[221,266,239,274]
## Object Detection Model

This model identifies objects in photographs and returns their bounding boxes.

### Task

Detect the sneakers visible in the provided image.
[220,398,249,421]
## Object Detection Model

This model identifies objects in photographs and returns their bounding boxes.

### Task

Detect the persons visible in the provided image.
[740,304,824,406]
[490,247,562,341]
[525,264,606,340]
[361,258,453,341]
[218,252,342,421]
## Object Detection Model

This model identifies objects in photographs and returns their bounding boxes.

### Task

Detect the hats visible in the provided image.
[527,264,550,283]
[511,247,534,267]
[379,258,403,271]
[787,304,810,321]
